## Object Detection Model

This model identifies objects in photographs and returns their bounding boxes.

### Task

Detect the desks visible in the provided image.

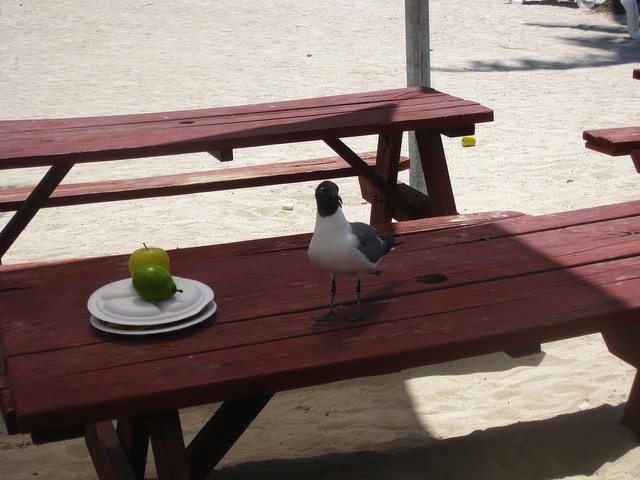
[1,199,640,438]
[2,86,495,213]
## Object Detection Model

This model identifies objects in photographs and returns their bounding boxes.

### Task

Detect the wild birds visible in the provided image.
[306,181,404,322]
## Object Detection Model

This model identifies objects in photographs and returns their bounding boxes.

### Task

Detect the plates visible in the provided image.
[87,276,214,326]
[89,301,218,335]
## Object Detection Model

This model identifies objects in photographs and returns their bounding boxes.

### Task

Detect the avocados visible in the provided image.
[132,263,183,300]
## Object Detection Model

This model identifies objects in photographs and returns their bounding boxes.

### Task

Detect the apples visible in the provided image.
[126,242,171,278]
[461,136,476,147]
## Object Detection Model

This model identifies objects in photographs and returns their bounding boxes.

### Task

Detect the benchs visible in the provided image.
[581,122,640,176]
[0,150,414,214]
